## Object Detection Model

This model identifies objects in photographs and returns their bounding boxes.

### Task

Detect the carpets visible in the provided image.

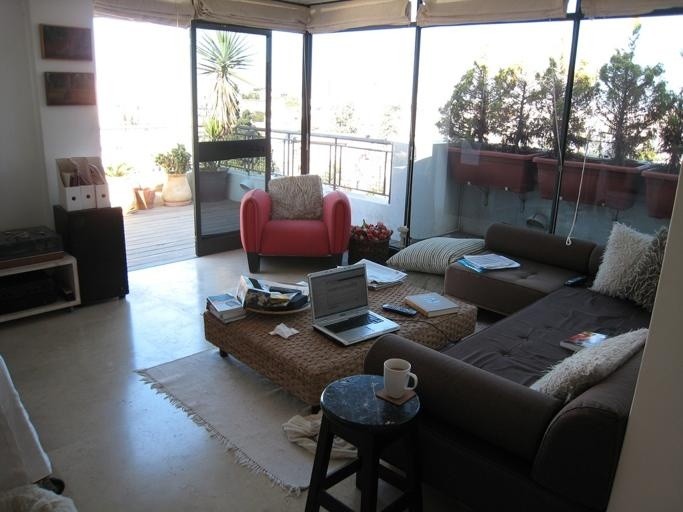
[133,308,505,497]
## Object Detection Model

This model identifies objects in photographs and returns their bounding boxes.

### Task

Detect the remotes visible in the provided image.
[382,304,417,316]
[269,286,301,296]
[564,275,589,286]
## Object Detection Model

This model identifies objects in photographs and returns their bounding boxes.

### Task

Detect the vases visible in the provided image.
[133,187,155,210]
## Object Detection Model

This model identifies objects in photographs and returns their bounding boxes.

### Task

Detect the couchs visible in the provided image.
[362,245,652,512]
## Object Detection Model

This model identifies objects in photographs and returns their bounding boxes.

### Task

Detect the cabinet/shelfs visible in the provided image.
[53,204,129,302]
[0,254,82,322]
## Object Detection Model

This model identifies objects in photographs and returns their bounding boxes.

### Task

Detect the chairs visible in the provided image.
[444,222,597,316]
[240,188,351,274]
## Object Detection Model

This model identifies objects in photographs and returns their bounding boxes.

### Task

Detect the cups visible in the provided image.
[382,358,418,399]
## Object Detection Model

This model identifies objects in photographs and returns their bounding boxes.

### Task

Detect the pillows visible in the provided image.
[617,226,669,312]
[588,222,654,297]
[529,328,649,405]
[385,236,485,275]
[269,175,323,220]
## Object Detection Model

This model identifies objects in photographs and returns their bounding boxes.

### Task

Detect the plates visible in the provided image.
[235,296,311,316]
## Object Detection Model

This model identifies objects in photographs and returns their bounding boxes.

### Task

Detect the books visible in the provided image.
[560,331,608,352]
[404,292,458,318]
[206,291,297,323]
[455,258,488,273]
[336,258,407,291]
[463,253,519,270]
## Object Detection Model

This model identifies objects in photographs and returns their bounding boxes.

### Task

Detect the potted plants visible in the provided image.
[199,114,229,203]
[155,143,193,206]
[104,161,134,214]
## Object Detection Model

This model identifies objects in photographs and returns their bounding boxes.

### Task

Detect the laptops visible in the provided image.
[308,263,400,345]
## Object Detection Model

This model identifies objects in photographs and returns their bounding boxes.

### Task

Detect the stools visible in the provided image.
[305,375,423,512]
